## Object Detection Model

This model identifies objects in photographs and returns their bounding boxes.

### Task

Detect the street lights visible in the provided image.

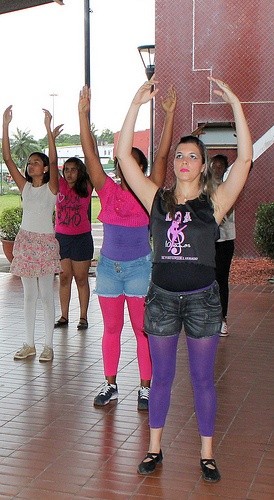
[137,45,155,175]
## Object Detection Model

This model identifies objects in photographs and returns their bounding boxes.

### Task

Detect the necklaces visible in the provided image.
[181,192,189,202]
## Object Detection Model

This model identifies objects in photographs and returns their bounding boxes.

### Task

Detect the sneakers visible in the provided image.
[39,346,54,362]
[14,342,36,359]
[137,386,151,413]
[94,380,119,406]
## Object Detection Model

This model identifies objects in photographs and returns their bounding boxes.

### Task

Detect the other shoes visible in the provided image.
[77,317,88,329]
[54,316,68,328]
[200,457,221,482]
[136,448,163,474]
[218,317,230,337]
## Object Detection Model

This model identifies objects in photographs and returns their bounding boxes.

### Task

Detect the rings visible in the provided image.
[222,82,225,85]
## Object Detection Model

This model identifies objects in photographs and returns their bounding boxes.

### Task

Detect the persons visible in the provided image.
[2,105,57,362]
[189,122,253,337]
[78,83,178,411]
[115,72,253,483]
[42,124,99,329]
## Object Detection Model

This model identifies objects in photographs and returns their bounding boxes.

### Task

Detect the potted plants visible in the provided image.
[0,205,56,264]
